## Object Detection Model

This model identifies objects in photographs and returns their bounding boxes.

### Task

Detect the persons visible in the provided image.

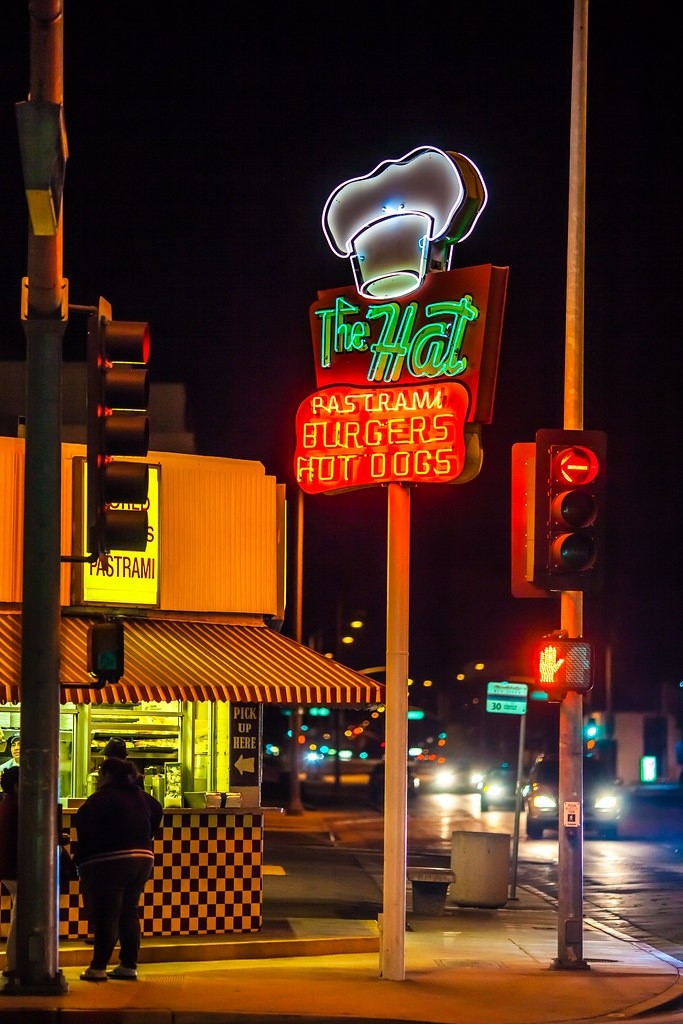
[0,766,70,973]
[75,758,162,982]
[0,736,144,791]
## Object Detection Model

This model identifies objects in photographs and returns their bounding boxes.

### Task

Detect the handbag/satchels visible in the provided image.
[59,843,77,880]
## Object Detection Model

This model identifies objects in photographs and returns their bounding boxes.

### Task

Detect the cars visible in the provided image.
[477,766,519,814]
[368,754,481,810]
[522,749,624,841]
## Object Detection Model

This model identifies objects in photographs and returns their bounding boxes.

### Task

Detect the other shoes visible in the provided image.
[85,938,94,944]
[2,970,16,977]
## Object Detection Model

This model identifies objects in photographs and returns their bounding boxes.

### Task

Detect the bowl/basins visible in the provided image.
[184,792,205,808]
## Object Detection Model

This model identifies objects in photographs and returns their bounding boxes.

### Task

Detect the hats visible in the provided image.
[10,733,20,744]
[99,737,129,758]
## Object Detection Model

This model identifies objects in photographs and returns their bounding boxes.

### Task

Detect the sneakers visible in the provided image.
[107,963,137,980]
[80,967,107,981]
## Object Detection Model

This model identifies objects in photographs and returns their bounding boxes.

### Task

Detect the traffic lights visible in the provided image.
[532,637,597,694]
[85,298,154,552]
[526,426,608,592]
[584,710,603,763]
